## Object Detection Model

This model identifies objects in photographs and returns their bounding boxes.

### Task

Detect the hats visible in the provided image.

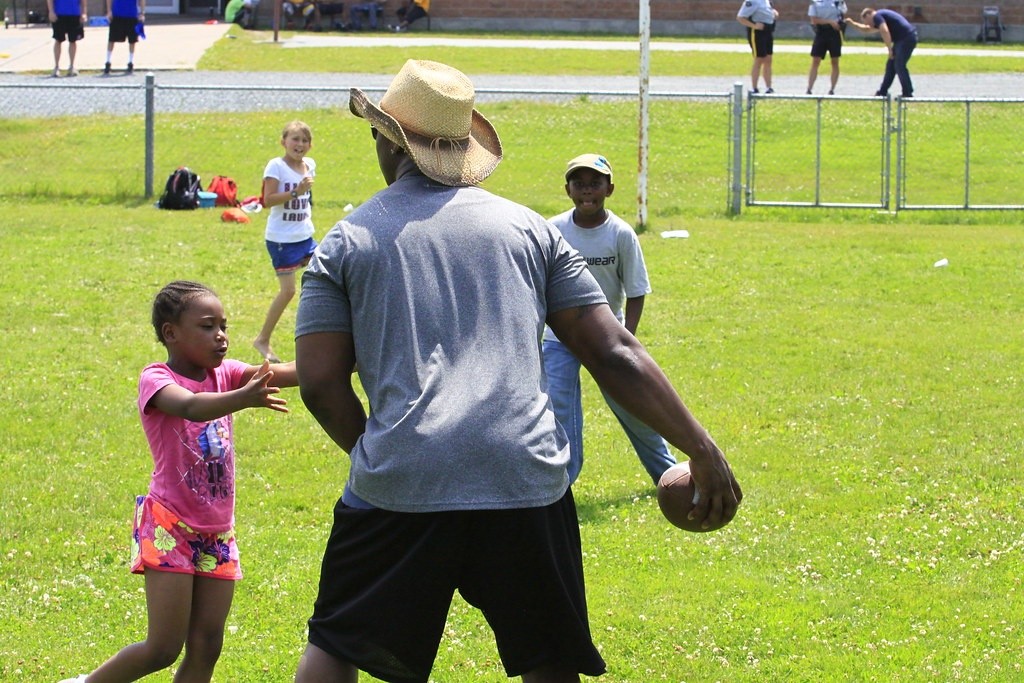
[349,59,504,186]
[564,154,613,185]
[136,22,145,39]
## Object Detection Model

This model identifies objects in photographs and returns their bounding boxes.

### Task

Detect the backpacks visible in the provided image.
[159,167,198,209]
[209,175,237,208]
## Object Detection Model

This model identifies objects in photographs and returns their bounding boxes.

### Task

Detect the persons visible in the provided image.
[845,8,919,98]
[806,0,848,95]
[293,60,744,683]
[104,0,145,75]
[311,0,351,31]
[282,0,316,31]
[736,0,778,95]
[225,0,253,29]
[57,281,358,683]
[351,0,387,31]
[47,0,85,79]
[253,118,318,363]
[387,0,430,32]
[540,153,676,487]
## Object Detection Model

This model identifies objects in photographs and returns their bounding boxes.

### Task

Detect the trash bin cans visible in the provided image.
[980,5,1001,45]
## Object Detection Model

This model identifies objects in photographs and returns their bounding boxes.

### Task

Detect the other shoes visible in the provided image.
[52,69,59,77]
[126,62,133,74]
[68,68,79,77]
[104,62,111,74]
[751,88,759,93]
[766,88,773,93]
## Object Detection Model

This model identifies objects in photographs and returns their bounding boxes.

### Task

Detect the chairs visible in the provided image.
[282,4,434,32]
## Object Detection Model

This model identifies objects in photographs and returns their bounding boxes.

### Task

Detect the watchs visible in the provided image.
[291,190,298,198]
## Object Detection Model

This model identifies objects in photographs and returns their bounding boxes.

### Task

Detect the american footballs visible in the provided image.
[656,459,738,533]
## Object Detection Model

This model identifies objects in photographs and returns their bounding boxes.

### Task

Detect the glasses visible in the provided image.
[370,123,380,140]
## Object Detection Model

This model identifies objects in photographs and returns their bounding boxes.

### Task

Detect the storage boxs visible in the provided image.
[197,191,218,206]
